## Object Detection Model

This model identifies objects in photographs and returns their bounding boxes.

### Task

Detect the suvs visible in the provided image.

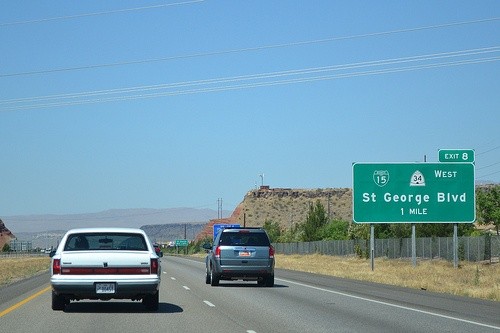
[202,226,275,286]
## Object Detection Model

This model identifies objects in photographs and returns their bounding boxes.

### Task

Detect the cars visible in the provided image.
[49,228,163,310]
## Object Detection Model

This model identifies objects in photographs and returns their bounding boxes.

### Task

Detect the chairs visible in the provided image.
[246,238,258,245]
[75,236,89,249]
